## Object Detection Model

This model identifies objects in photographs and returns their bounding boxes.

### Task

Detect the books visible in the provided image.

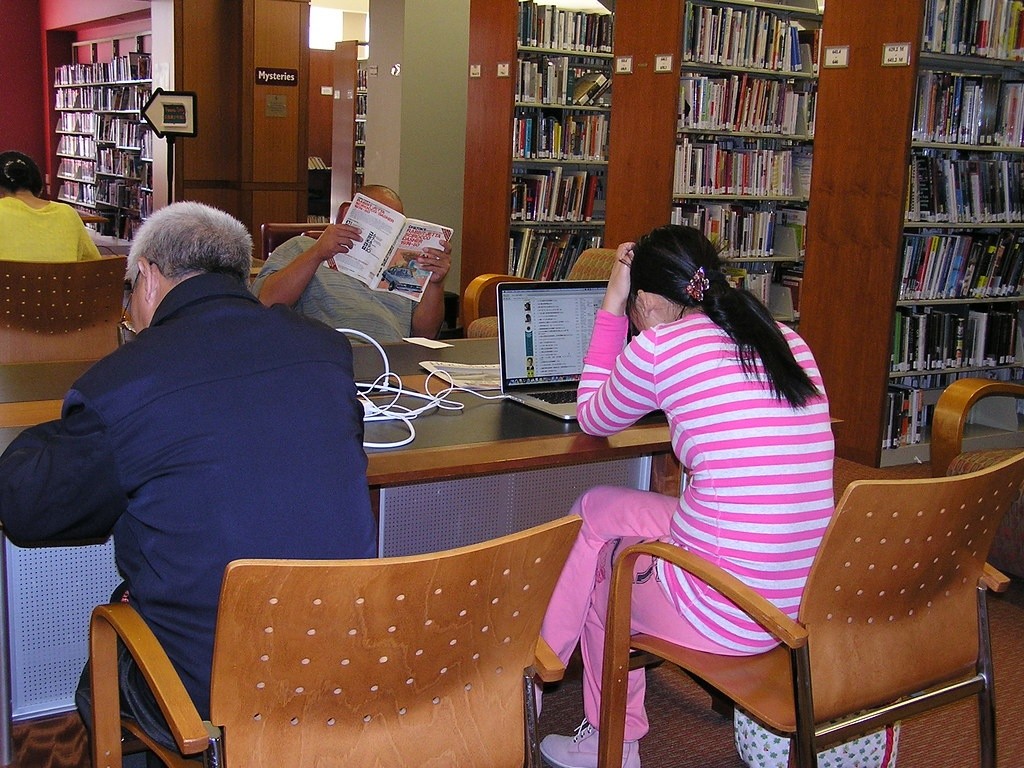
[307,156,332,170]
[98,114,153,242]
[353,69,368,196]
[511,167,604,222]
[54,52,152,85]
[55,84,152,110]
[509,1,613,53]
[306,215,330,223]
[516,54,614,106]
[513,107,612,161]
[509,227,604,281]
[672,0,822,333]
[321,192,455,302]
[882,0,1024,449]
[58,111,97,230]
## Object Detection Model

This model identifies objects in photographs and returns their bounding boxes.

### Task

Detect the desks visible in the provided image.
[1,336,844,768]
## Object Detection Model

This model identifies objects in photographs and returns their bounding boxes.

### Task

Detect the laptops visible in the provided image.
[496,279,632,420]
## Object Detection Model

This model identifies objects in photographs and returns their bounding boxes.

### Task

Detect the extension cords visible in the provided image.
[358,391,440,417]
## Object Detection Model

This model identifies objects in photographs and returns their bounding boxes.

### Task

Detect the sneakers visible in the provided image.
[540,717,640,768]
[534,681,543,719]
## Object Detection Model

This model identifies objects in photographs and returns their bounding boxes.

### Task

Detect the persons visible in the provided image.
[1,200,377,768]
[249,185,452,338]
[527,227,835,768]
[0,151,104,261]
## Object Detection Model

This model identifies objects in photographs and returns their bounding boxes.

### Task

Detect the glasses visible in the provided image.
[120,261,153,334]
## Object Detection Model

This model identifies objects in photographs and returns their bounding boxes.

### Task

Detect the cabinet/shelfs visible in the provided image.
[343,34,374,203]
[48,36,156,241]
[464,0,1024,485]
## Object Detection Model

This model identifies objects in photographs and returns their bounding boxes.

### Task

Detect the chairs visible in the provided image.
[596,448,1023,768]
[1,257,128,364]
[86,510,583,768]
